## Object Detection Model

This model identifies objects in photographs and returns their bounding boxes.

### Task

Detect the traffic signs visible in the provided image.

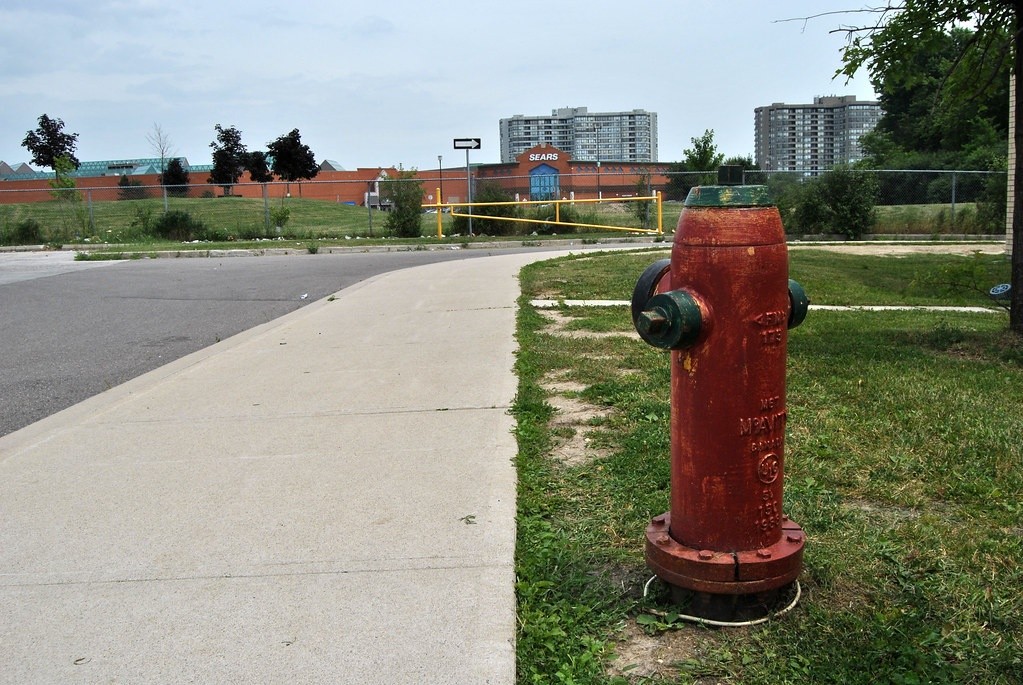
[453,137,481,149]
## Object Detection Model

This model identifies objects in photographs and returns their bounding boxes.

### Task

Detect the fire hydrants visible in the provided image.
[631,159,817,625]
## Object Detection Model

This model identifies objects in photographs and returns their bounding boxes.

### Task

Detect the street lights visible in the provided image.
[436,153,445,205]
